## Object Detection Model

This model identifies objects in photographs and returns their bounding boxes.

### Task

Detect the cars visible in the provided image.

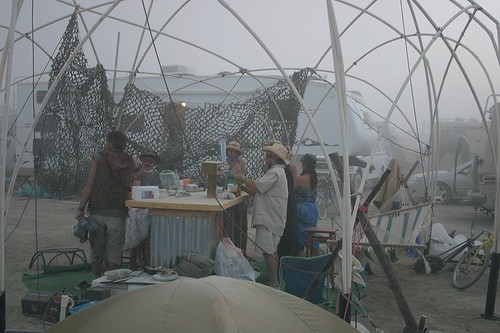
[407,160,482,206]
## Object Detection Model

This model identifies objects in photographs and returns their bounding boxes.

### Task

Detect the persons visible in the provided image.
[226,141,247,254]
[268,140,320,257]
[237,143,288,286]
[76,131,134,278]
[133,153,164,189]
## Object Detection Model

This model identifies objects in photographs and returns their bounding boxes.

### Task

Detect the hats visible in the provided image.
[139,147,160,162]
[262,144,290,165]
[300,154,316,166]
[225,141,244,156]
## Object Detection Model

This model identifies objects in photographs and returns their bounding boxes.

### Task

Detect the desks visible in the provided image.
[126,191,253,273]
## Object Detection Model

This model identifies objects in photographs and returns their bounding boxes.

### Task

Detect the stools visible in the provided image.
[121,240,145,272]
[306,226,339,276]
[30,248,88,269]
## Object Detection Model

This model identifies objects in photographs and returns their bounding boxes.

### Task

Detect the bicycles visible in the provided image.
[414,208,494,291]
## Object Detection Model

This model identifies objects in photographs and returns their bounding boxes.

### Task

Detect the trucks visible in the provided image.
[478,94,500,211]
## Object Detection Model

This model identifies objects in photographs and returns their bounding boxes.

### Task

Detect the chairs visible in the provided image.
[276,252,334,308]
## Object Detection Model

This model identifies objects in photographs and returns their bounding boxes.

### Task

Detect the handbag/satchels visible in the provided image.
[213,218,256,282]
[123,208,149,250]
[173,252,214,278]
